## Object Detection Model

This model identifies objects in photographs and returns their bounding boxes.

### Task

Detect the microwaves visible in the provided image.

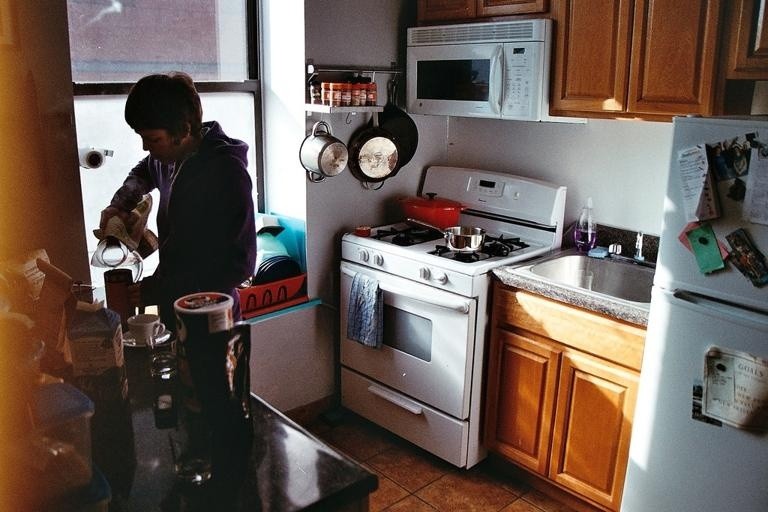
[405,19,588,125]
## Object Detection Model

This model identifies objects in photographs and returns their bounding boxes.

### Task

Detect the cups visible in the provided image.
[149,351,179,418]
[127,314,165,344]
[104,268,145,334]
[168,421,215,483]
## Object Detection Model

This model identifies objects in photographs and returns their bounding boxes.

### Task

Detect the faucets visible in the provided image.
[636,230,646,260]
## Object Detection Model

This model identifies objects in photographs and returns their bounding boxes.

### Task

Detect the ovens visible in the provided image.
[340,262,490,471]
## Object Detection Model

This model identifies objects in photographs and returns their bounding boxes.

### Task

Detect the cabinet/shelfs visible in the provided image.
[479,277,646,512]
[414,0,549,24]
[551,1,767,120]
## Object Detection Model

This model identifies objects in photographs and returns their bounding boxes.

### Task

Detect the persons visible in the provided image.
[97,69,258,332]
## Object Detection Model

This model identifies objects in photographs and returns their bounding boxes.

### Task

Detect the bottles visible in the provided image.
[309,77,376,108]
[173,291,235,362]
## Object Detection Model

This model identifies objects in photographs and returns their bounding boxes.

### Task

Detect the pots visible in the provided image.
[300,121,349,183]
[348,112,401,191]
[403,214,486,255]
[368,78,419,168]
[394,192,468,232]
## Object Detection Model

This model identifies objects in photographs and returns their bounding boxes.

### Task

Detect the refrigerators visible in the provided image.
[620,113,768,512]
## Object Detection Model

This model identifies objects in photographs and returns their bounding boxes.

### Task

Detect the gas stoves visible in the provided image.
[342,167,570,276]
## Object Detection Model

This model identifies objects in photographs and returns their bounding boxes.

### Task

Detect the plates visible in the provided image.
[122,329,172,347]
[355,226,370,237]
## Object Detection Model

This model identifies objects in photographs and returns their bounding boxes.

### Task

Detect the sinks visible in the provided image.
[511,248,656,307]
